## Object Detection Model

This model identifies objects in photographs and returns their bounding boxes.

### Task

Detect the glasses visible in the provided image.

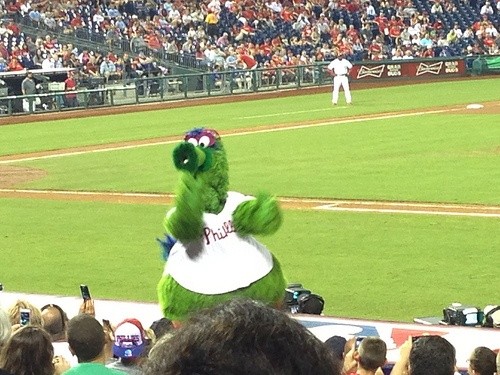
[40,304,65,331]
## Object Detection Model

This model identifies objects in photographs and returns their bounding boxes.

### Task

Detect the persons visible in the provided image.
[0,299,500,375]
[327,52,353,105]
[0,0,500,118]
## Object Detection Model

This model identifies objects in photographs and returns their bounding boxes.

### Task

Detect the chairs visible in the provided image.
[155,0,500,95]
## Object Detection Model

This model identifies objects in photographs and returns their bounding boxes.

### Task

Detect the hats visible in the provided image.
[115,319,147,357]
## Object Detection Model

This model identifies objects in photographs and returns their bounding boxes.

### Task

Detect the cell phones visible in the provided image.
[20,309,30,328]
[80,285,91,302]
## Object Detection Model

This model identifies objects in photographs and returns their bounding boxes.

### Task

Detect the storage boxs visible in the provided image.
[443,304,485,326]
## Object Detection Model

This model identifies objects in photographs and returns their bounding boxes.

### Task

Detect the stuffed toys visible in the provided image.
[157,126,288,327]
